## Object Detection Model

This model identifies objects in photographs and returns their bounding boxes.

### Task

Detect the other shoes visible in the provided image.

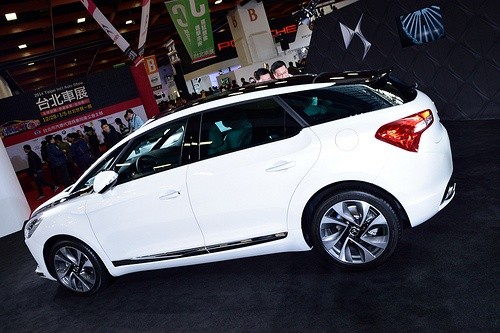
[35,195,45,200]
[53,186,59,192]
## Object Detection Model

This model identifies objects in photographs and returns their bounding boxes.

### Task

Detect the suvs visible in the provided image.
[23,72,456,301]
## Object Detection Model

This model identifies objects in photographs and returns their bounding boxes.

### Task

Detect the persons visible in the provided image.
[21,108,145,201]
[158,57,309,116]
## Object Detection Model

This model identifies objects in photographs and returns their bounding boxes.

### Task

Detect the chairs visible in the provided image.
[208,111,253,155]
